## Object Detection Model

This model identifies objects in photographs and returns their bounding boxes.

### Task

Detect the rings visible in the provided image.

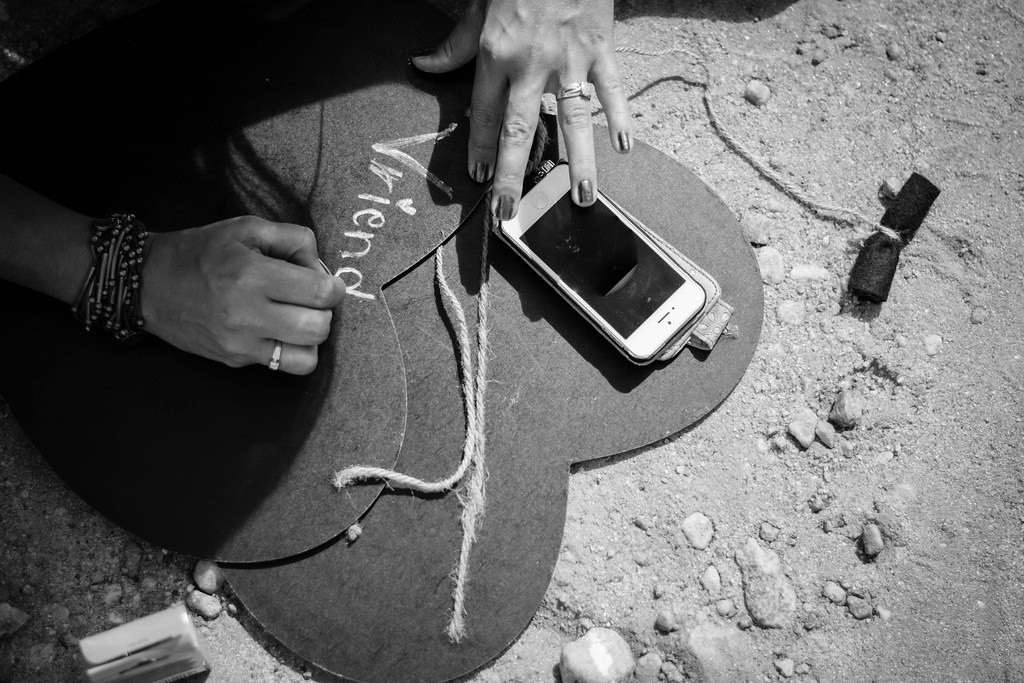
[269,341,282,371]
[557,82,591,100]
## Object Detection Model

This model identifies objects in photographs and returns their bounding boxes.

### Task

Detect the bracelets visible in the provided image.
[70,214,150,340]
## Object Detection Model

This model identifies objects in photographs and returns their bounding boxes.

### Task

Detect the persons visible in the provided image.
[0,0,634,376]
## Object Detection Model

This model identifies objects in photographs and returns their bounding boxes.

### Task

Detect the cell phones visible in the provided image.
[494,161,708,365]
